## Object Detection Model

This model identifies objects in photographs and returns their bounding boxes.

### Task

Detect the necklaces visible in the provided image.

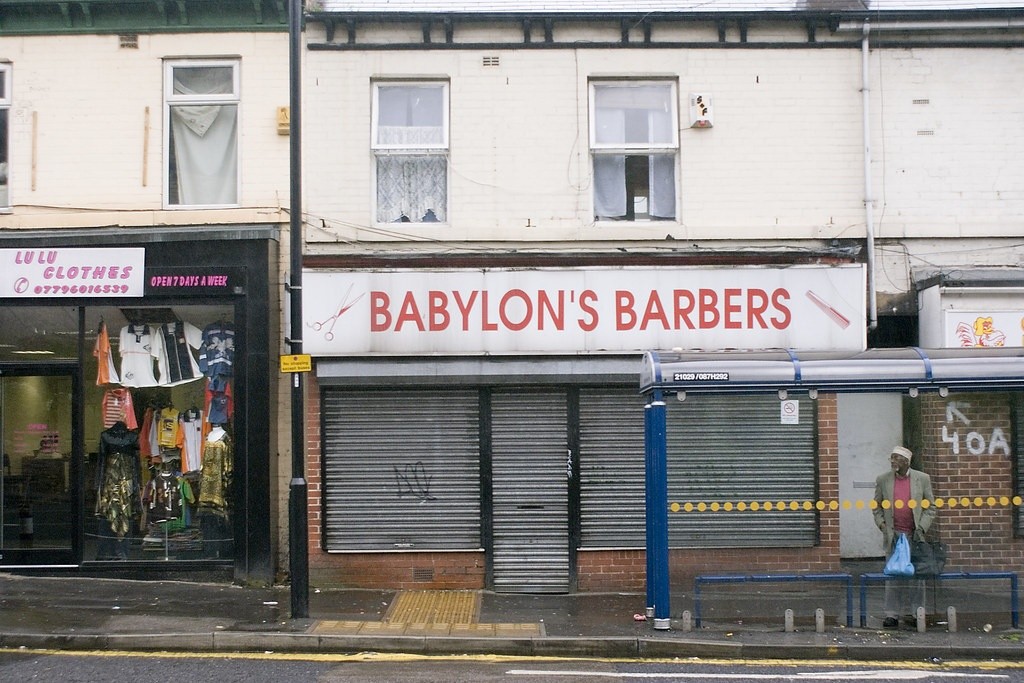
[96,421,142,542]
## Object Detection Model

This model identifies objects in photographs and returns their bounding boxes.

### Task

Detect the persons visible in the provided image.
[872,446,936,629]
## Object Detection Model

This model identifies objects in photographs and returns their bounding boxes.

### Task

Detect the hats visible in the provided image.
[890,445,913,463]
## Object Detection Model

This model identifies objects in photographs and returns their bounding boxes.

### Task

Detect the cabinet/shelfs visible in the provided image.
[2,448,100,513]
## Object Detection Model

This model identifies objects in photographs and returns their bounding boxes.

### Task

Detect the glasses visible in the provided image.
[888,457,898,460]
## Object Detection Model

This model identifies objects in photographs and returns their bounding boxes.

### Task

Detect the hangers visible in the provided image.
[96,312,238,421]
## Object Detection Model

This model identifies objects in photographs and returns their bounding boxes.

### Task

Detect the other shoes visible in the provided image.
[907,617,918,627]
[883,617,898,628]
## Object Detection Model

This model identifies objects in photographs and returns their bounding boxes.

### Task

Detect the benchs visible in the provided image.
[858,570,1017,627]
[693,571,853,627]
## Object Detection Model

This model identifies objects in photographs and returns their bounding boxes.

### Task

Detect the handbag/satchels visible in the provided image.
[883,533,914,576]
[911,539,947,574]
